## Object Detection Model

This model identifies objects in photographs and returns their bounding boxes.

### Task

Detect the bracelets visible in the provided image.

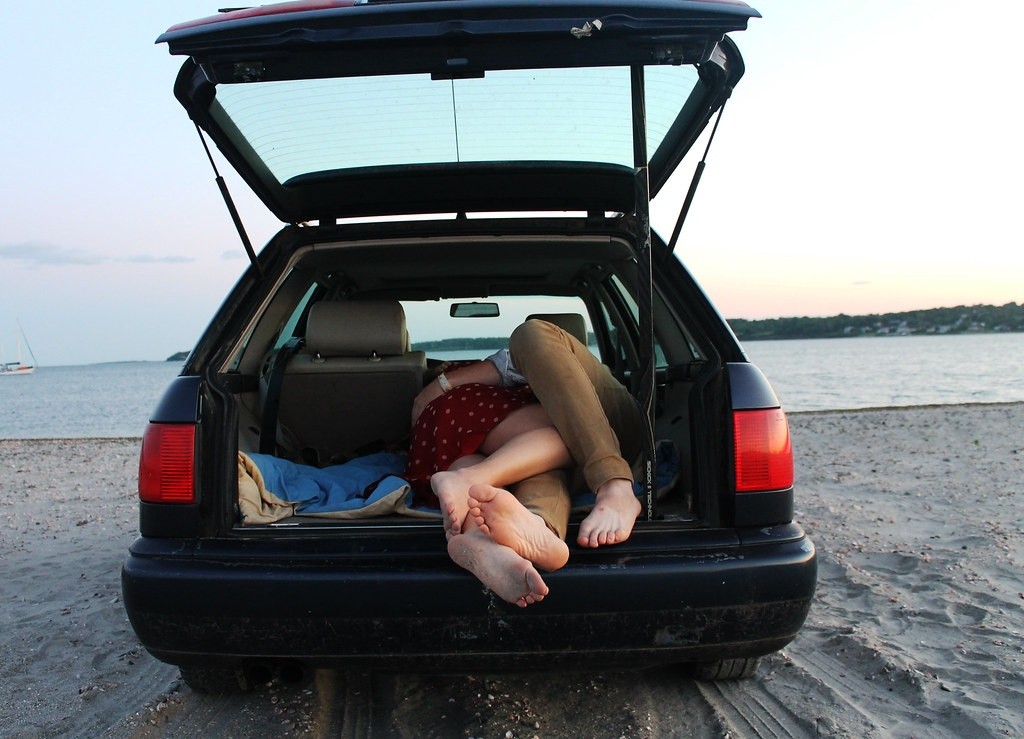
[437,372,453,393]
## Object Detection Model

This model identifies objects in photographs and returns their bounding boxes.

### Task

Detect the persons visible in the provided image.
[404,319,648,608]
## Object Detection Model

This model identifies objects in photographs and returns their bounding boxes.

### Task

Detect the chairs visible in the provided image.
[277,298,427,454]
[525,313,588,352]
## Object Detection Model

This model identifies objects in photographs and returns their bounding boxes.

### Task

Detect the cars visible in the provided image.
[121,0,817,695]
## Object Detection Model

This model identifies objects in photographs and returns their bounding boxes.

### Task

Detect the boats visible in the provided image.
[0,320,39,375]
